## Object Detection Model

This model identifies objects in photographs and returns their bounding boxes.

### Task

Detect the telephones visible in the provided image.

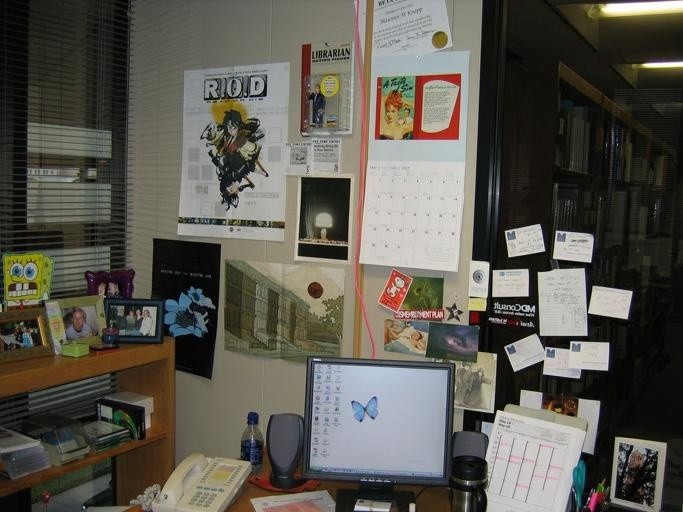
[151,453,253,512]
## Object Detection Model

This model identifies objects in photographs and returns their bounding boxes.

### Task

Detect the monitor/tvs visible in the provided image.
[302,355,455,512]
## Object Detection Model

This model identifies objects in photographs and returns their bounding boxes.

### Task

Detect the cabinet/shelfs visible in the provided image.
[0,340,176,511]
[487,29,682,450]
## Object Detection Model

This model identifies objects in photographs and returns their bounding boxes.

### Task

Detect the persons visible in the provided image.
[97,283,106,300]
[385,319,398,346]
[402,329,426,350]
[401,108,413,141]
[307,85,326,128]
[107,280,122,298]
[379,89,413,141]
[64,308,99,339]
[110,305,152,336]
[2,321,42,351]
[395,116,408,141]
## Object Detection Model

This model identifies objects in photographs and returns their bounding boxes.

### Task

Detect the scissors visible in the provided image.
[573,460,586,512]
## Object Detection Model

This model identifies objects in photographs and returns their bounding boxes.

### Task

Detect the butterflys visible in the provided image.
[351,397,378,422]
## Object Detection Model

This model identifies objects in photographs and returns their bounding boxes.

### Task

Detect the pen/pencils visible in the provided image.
[581,477,612,512]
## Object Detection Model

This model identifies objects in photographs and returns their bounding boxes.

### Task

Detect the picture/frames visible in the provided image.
[608,435,667,512]
[44,294,106,347]
[0,308,56,364]
[103,297,164,346]
[83,267,136,298]
[293,171,354,266]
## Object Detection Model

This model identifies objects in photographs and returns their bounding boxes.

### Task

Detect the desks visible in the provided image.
[127,444,453,511]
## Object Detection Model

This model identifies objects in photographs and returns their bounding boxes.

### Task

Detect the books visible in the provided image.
[0,425,40,453]
[538,100,669,397]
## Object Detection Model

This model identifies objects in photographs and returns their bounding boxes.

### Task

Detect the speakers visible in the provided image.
[266,413,308,489]
[451,431,489,460]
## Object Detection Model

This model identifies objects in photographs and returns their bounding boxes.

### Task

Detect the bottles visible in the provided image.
[240,412,264,481]
[449,454,488,512]
